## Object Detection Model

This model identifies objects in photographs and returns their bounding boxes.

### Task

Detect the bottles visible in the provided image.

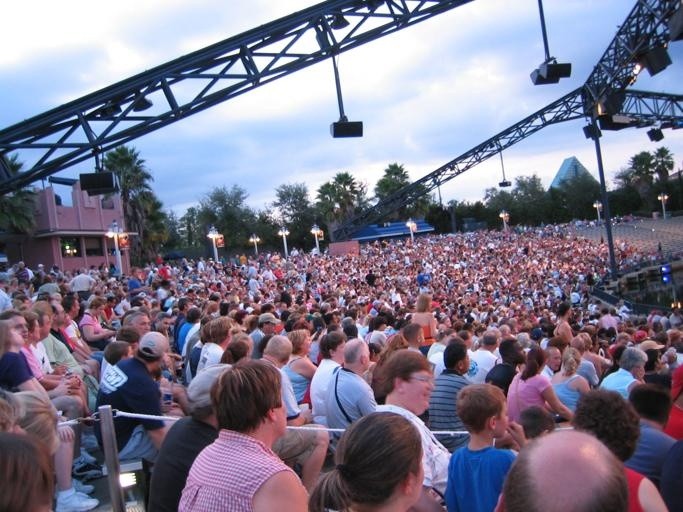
[176,358,182,377]
[160,370,174,408]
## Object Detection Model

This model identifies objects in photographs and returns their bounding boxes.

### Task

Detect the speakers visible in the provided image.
[80,171,115,188]
[529,70,560,84]
[540,63,571,79]
[331,122,363,138]
[87,176,120,196]
[670,7,683,42]
[647,129,663,141]
[583,126,593,138]
[641,47,672,76]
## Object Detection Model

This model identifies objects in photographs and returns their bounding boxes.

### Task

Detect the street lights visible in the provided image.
[498,209,509,229]
[277,227,290,258]
[583,12,682,146]
[249,232,261,254]
[207,222,220,262]
[104,220,125,278]
[310,222,320,249]
[404,217,416,241]
[656,192,668,220]
[592,201,602,220]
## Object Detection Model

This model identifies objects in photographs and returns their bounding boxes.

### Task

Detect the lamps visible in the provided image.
[527,0,574,92]
[645,122,664,142]
[320,50,363,140]
[635,37,674,77]
[79,148,132,200]
[326,5,350,32]
[581,120,602,140]
[103,88,153,116]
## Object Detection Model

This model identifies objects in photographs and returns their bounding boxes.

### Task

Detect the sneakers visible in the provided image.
[56,489,99,511]
[52,478,95,495]
[72,426,104,480]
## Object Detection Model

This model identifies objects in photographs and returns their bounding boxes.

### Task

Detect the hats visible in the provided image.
[138,331,169,358]
[258,312,282,326]
[186,363,232,410]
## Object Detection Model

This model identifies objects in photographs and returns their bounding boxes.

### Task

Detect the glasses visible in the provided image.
[403,374,434,387]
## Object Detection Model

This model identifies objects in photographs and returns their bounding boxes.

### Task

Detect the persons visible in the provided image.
[0,213,683,512]
